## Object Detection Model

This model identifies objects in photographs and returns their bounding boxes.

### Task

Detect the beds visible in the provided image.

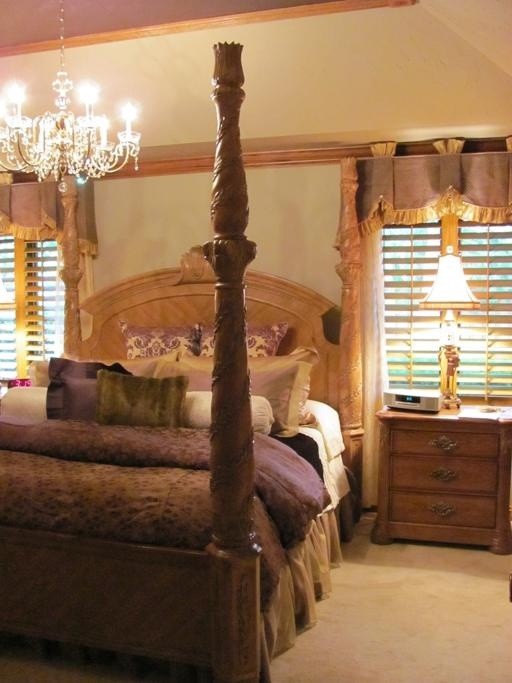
[0,41,364,683]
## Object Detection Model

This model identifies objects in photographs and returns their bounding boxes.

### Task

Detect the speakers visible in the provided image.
[384,389,442,412]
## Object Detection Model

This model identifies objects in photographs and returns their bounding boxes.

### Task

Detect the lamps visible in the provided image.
[417,245,481,410]
[0,0,142,193]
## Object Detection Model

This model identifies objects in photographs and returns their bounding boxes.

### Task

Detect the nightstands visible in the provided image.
[371,405,512,555]
